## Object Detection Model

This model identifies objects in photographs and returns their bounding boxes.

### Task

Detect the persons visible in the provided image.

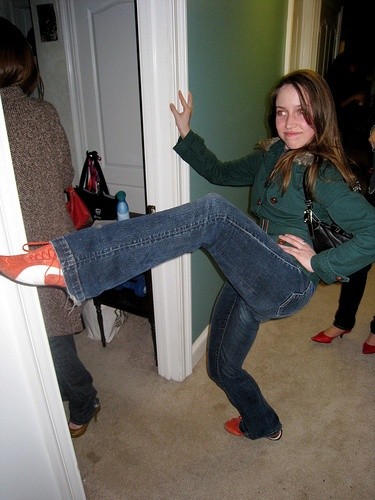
[0,69,375,441]
[0,16,99,437]
[311,97,375,354]
[323,52,365,156]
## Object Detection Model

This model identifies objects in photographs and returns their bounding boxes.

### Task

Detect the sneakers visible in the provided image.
[0,240,67,288]
[224,417,283,441]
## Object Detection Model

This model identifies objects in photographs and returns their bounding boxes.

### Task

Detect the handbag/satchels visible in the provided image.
[62,184,92,230]
[80,300,127,343]
[302,160,360,255]
[73,151,117,220]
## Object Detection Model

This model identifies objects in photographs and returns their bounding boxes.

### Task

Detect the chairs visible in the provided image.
[93,205,157,367]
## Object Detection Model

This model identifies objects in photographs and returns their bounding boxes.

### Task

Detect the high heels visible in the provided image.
[68,403,101,438]
[310,328,351,343]
[362,342,375,354]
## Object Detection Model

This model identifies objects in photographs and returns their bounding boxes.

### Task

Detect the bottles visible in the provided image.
[116,191,129,221]
